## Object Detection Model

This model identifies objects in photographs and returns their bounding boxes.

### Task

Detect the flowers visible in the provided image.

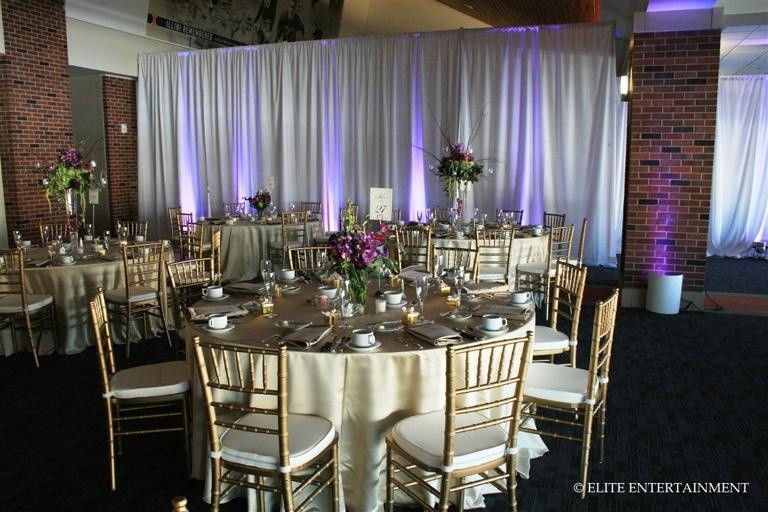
[29,133,109,215]
[242,189,273,214]
[410,112,496,197]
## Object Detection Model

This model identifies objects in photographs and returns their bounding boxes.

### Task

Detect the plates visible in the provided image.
[202,293,230,301]
[346,339,382,351]
[476,324,510,335]
[202,324,235,334]
[504,298,532,307]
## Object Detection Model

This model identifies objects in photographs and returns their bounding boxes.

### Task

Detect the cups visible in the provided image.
[9,223,172,266]
[208,314,227,329]
[352,329,375,347]
[483,314,508,331]
[511,291,530,304]
[201,286,223,298]
[223,198,316,226]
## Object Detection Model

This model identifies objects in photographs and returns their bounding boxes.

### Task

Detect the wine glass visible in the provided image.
[412,204,546,242]
[258,246,482,331]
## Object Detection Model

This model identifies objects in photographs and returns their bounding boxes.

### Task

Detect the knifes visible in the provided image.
[321,334,350,354]
[453,325,487,343]
